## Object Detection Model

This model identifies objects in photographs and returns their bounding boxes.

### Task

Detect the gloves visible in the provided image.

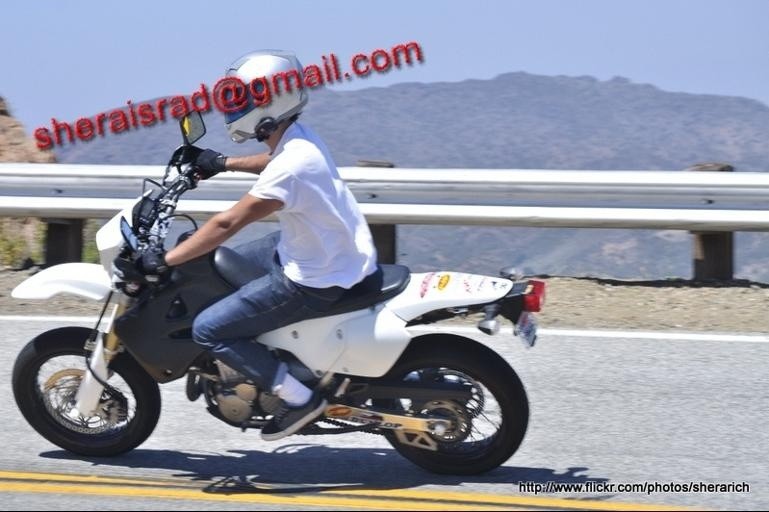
[138,250,170,285]
[195,148,226,179]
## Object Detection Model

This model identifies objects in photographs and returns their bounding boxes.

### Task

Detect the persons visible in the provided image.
[136,46,380,444]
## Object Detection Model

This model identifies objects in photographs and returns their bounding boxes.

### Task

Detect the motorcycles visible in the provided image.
[9,112,549,479]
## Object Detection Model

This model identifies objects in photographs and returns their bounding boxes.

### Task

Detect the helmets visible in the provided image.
[224,51,307,144]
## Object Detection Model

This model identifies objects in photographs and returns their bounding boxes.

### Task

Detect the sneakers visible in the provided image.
[262,392,326,440]
